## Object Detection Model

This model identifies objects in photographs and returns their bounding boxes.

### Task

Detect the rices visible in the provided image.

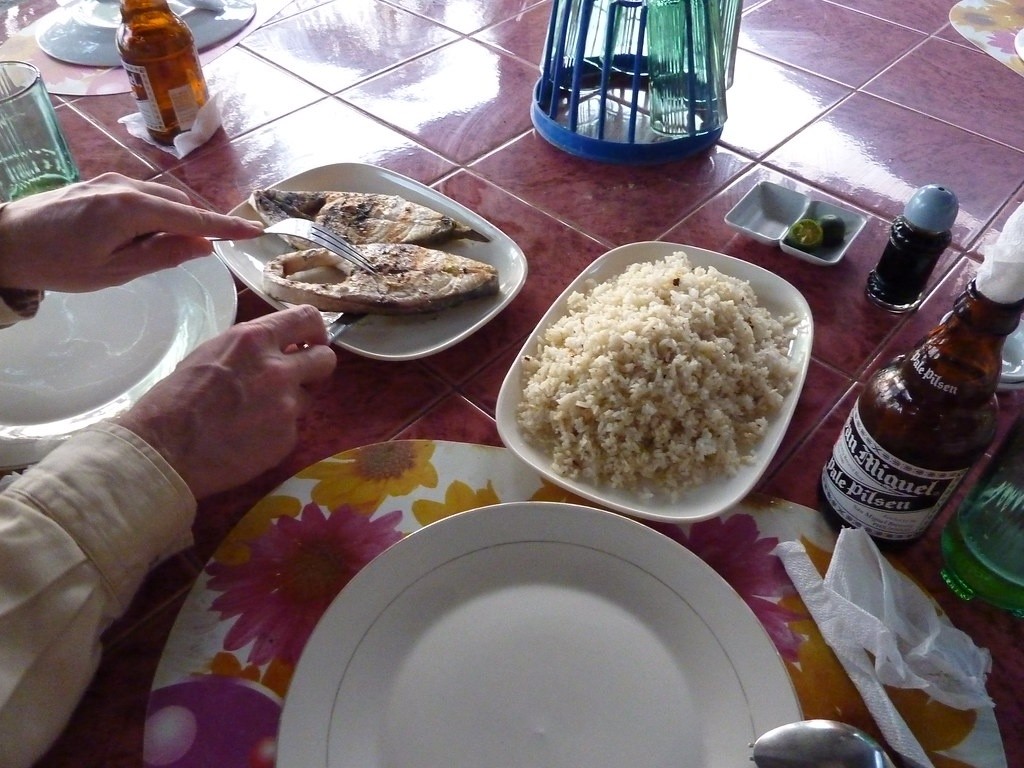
[516,248,800,500]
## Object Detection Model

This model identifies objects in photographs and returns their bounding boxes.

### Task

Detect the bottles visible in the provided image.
[819,276,1024,550]
[864,184,959,314]
[940,411,1024,620]
[114,0,211,147]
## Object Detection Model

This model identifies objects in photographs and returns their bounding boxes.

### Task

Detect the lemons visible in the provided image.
[785,214,846,252]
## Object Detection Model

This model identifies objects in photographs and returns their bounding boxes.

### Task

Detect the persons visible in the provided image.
[1,172,336,768]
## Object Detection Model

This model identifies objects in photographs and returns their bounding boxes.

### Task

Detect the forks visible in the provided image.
[204,218,376,276]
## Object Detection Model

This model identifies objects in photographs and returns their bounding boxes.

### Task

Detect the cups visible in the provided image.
[717,0,743,91]
[0,61,79,204]
[646,0,728,138]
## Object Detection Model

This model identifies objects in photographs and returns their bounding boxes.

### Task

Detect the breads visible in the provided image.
[260,241,499,319]
[248,188,494,252]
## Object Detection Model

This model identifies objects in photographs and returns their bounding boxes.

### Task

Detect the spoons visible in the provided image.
[753,718,897,768]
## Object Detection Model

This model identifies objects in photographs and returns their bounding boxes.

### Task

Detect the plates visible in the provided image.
[139,439,1009,768]
[36,0,257,68]
[495,241,814,524]
[274,501,804,767]
[212,161,529,363]
[0,250,237,472]
[725,181,811,247]
[779,201,868,268]
